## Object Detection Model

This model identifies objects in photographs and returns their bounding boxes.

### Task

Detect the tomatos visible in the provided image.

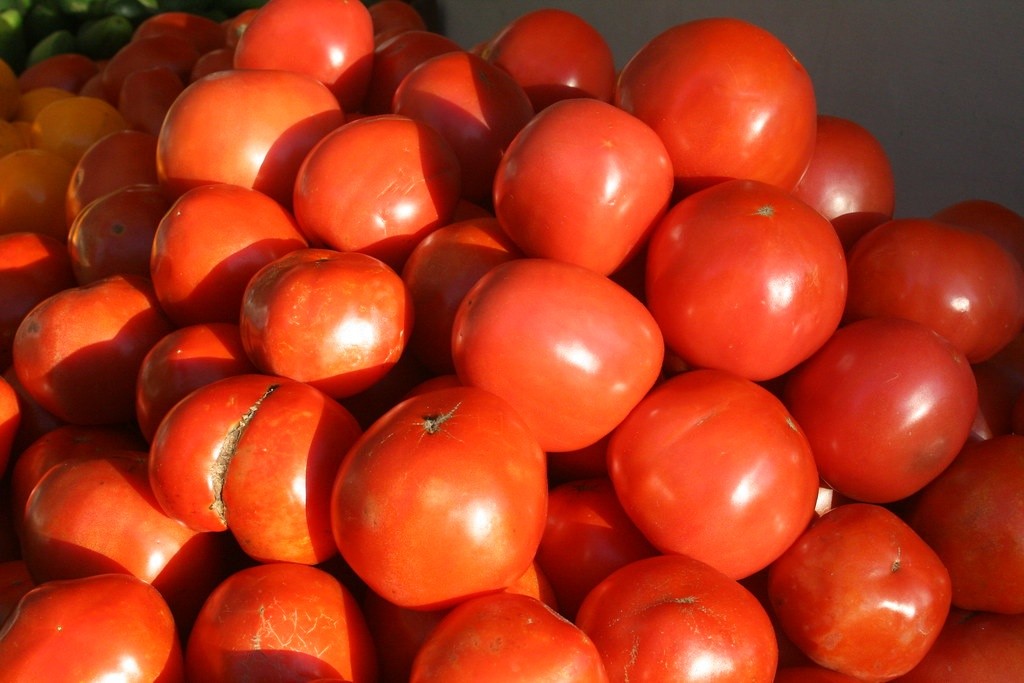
[0,0,1024,683]
